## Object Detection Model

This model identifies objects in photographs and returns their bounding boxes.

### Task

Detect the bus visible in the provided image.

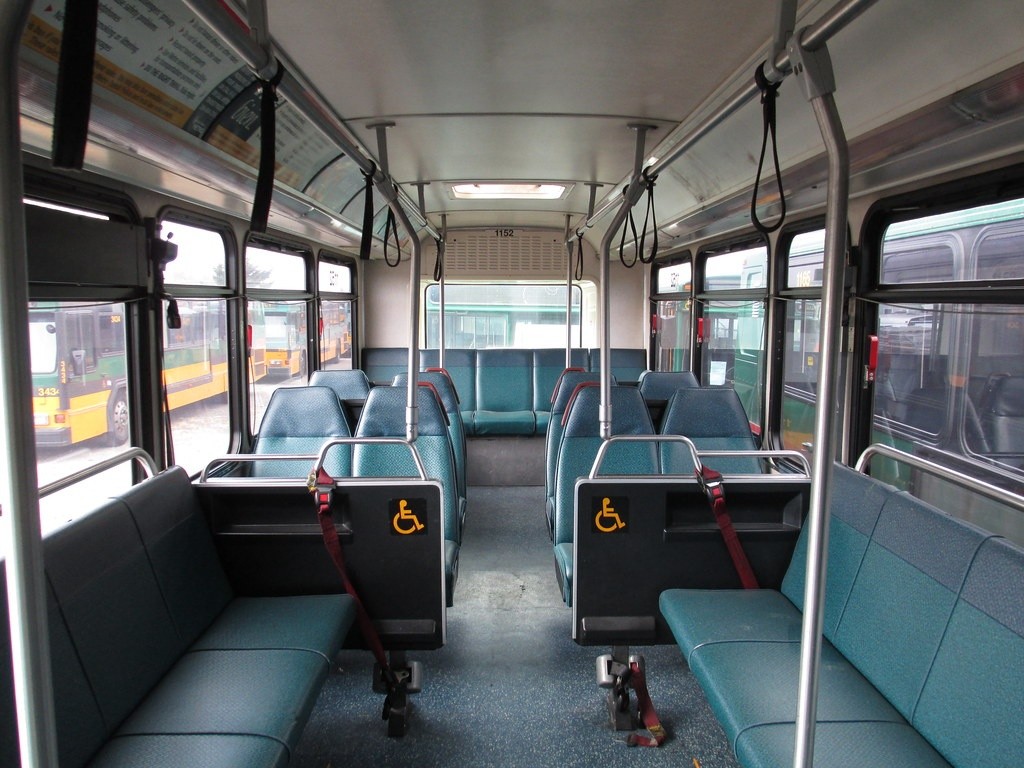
[746,194,1024,550]
[265,301,349,378]
[29,301,268,451]
[657,272,741,386]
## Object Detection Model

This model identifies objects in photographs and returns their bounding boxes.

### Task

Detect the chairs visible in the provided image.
[658,384,766,476]
[552,381,663,608]
[544,366,617,543]
[590,346,647,388]
[687,490,1009,762]
[0,548,293,768]
[43,498,330,758]
[349,381,463,609]
[473,347,537,436]
[636,371,702,435]
[658,459,900,673]
[734,535,1024,768]
[307,369,371,435]
[418,348,477,438]
[109,463,361,670]
[390,366,469,538]
[532,348,590,437]
[361,345,410,387]
[246,384,353,479]
